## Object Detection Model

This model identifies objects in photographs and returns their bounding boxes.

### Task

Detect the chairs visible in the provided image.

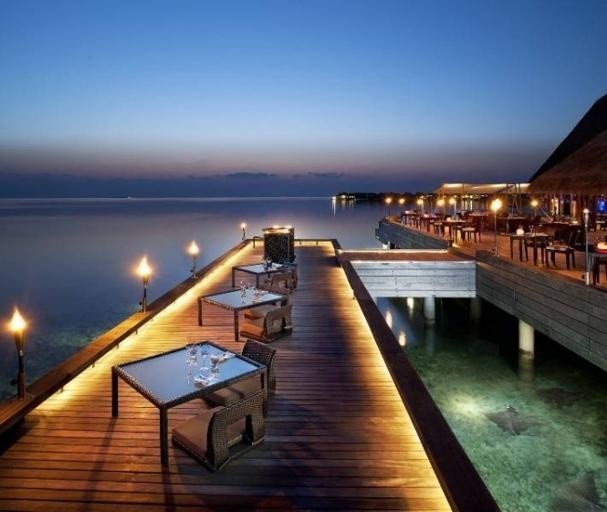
[204,339,277,405]
[239,304,294,343]
[232,261,297,295]
[393,199,607,290]
[172,389,267,471]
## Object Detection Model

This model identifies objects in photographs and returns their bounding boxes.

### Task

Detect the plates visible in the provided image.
[525,233,531,234]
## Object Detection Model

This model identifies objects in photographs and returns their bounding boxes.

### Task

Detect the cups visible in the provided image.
[262,256,272,270]
[239,280,261,303]
[184,336,219,377]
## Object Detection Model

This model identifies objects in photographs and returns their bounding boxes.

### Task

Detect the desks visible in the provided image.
[197,285,287,342]
[111,340,267,463]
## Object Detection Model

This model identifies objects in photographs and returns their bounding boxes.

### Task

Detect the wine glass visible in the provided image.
[528,225,533,234]
